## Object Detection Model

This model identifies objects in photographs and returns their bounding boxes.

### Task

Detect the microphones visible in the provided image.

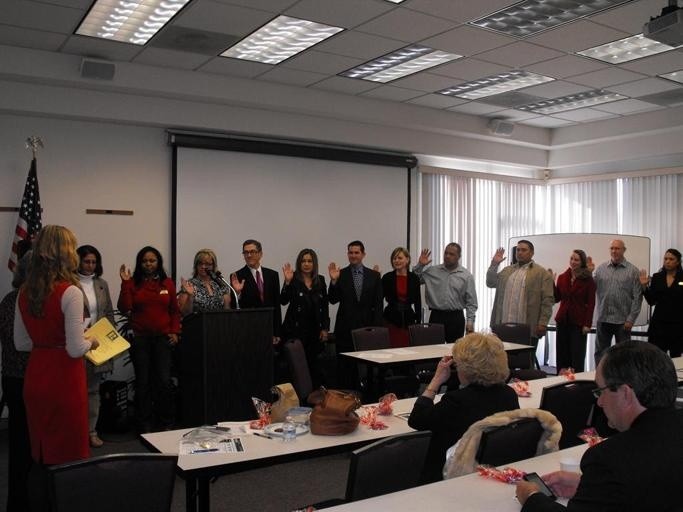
[206,269,224,290]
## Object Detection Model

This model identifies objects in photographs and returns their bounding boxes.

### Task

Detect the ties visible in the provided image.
[354,269,361,300]
[256,269,263,304]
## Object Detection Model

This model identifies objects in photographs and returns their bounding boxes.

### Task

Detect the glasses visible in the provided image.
[197,261,214,267]
[591,381,632,400]
[242,249,260,255]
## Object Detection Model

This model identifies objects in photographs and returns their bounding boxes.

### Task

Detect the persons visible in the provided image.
[586,239,643,366]
[640,247,683,356]
[409,330,520,487]
[13,225,96,464]
[74,244,116,449]
[373,247,421,330]
[230,240,282,345]
[179,249,228,313]
[280,249,331,345]
[117,246,180,432]
[515,340,683,511]
[413,243,478,344]
[328,240,383,354]
[0,250,39,511]
[485,241,555,371]
[548,250,595,375]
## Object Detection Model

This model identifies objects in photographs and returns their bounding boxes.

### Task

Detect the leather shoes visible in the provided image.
[137,431,150,436]
[162,427,169,430]
[90,433,105,448]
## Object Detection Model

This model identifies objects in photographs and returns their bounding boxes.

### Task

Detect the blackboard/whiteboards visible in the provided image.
[506,232,651,328]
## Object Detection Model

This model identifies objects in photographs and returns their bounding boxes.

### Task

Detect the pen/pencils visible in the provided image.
[253,432,272,440]
[192,448,218,452]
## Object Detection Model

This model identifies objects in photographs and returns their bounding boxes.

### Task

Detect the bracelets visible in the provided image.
[426,387,437,394]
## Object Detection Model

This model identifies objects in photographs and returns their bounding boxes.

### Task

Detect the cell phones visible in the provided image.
[524,472,557,501]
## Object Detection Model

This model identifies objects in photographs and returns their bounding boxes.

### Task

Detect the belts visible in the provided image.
[497,321,528,330]
[432,308,464,314]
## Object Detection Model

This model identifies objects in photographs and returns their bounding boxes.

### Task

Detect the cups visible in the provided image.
[282,421,295,444]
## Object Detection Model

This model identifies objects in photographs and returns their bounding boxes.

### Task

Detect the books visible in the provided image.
[83,317,131,365]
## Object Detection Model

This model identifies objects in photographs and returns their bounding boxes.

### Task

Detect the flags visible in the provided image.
[8,148,42,273]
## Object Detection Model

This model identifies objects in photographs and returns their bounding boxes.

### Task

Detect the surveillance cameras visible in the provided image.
[543,170,550,179]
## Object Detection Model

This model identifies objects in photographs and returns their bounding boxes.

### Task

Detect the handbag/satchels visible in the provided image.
[307,387,360,436]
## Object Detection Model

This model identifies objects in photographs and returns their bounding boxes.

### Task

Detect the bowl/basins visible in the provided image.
[288,407,310,422]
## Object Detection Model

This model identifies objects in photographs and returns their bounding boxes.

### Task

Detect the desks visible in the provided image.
[369,369,597,432]
[312,438,610,512]
[339,341,536,368]
[140,400,420,512]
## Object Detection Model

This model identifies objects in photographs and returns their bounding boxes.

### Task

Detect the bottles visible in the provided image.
[282,416,296,443]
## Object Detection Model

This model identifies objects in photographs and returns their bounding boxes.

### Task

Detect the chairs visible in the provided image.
[46,452,178,511]
[475,417,544,468]
[493,321,547,383]
[346,429,433,502]
[408,323,445,346]
[351,327,390,390]
[539,380,599,450]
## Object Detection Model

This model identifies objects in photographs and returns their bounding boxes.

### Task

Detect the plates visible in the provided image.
[265,423,307,437]
